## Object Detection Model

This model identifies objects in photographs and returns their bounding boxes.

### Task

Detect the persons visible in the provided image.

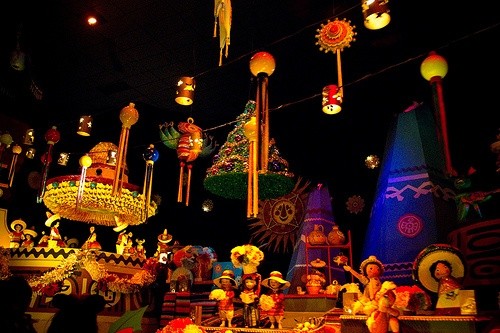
[0,276,104,333]
[212,242,465,333]
[7,212,174,263]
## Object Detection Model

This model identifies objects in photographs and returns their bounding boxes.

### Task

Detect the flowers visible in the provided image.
[209,289,226,301]
[259,294,275,311]
[231,245,264,268]
[157,317,235,333]
[393,285,432,313]
[0,247,160,297]
